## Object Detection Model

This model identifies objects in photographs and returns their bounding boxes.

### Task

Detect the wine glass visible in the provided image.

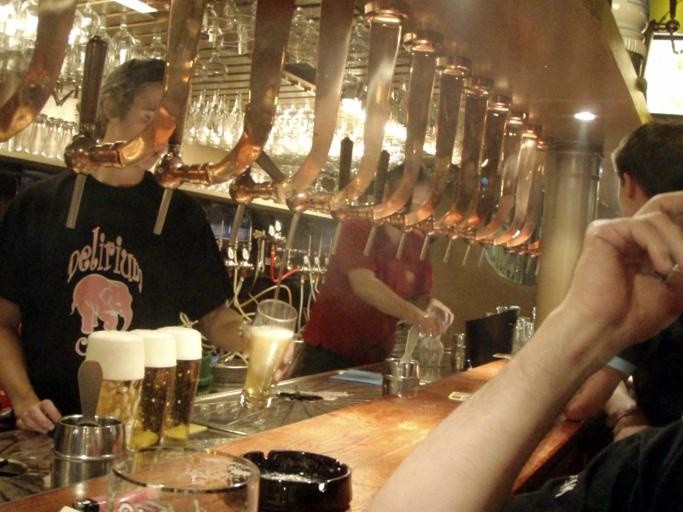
[72,2,320,166]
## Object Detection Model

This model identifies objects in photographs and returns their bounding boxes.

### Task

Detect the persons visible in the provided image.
[365,187,682,510]
[2,57,296,435]
[283,162,454,380]
[560,124,681,477]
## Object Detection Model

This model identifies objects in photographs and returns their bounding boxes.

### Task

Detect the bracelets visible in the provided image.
[239,323,249,341]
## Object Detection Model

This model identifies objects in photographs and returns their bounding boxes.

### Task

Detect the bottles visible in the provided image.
[418,329,444,368]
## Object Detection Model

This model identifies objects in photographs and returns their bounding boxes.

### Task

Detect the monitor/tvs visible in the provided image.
[641,34,683,119]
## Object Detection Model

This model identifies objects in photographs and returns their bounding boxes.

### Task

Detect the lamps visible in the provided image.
[634,0,683,91]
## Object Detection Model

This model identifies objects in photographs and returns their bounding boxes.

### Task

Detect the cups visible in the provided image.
[240,299,298,411]
[104,445,262,511]
[84,327,203,455]
[8,112,78,160]
